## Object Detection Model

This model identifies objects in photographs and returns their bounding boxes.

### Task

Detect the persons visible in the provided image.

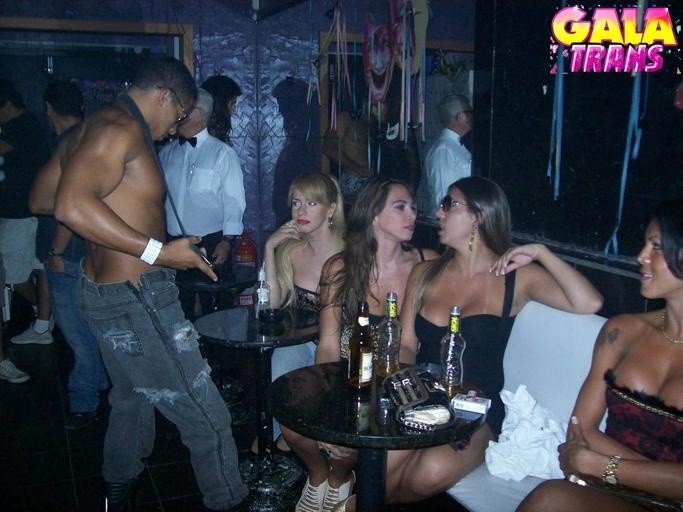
[0,86,57,346]
[268,175,442,512]
[511,196,683,512]
[21,78,110,424]
[331,177,604,512]
[322,73,378,195]
[28,54,296,512]
[423,93,475,223]
[158,88,249,316]
[367,76,421,194]
[249,171,350,459]
[1,257,31,385]
[196,75,244,146]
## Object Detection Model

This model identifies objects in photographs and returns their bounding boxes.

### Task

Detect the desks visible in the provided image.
[195,306,320,511]
[258,359,491,512]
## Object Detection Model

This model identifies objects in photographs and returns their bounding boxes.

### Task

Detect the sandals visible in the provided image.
[295,466,333,512]
[321,469,356,512]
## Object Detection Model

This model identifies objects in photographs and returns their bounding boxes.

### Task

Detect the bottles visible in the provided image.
[345,300,375,392]
[437,305,468,397]
[253,266,275,320]
[373,291,403,379]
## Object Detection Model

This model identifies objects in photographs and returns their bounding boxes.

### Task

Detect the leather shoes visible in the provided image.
[333,493,357,512]
[248,444,258,457]
[271,432,292,457]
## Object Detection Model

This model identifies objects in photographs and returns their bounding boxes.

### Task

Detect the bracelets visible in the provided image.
[46,249,64,257]
[139,237,163,267]
[221,236,236,246]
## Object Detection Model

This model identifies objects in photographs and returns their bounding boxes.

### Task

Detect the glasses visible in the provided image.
[156,85,188,124]
[455,110,475,120]
[441,195,473,212]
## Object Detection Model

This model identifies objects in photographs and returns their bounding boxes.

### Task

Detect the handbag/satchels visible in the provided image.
[382,367,456,432]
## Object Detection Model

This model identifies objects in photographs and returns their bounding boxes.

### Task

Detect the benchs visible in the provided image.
[254,215,617,510]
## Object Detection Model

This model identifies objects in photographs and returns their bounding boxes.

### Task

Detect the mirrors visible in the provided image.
[252,1,679,266]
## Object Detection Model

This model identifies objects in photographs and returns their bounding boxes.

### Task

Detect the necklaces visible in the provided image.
[661,307,683,346]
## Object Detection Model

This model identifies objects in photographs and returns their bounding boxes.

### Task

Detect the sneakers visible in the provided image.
[31,304,56,334]
[10,322,54,345]
[0,358,30,384]
[64,412,98,430]
[103,480,136,512]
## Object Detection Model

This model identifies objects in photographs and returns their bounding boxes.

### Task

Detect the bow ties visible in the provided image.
[179,136,197,147]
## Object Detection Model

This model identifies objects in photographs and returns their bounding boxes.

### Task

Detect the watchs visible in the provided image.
[601,454,622,489]
